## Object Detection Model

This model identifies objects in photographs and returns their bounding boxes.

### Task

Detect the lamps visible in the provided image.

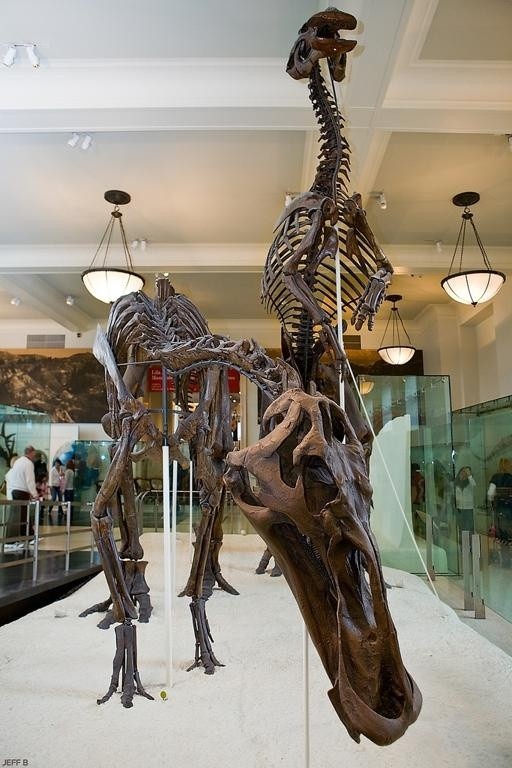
[359,374,375,395]
[436,190,507,309]
[80,189,144,306]
[377,293,417,367]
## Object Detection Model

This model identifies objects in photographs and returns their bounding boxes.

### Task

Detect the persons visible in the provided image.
[6,443,77,538]
[411,463,424,537]
[449,464,476,554]
[484,457,511,572]
